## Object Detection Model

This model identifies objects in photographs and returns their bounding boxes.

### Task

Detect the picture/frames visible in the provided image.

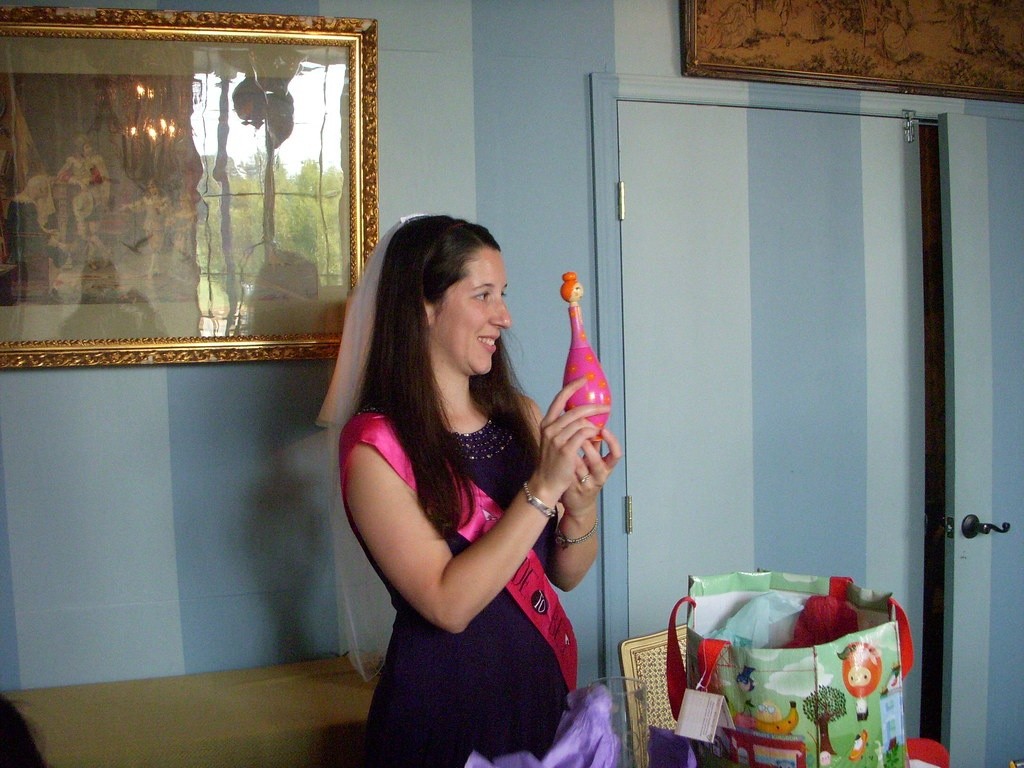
[0,6,379,369]
[680,0,1024,104]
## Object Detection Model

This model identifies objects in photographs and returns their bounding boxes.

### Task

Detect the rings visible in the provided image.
[580,473,591,483]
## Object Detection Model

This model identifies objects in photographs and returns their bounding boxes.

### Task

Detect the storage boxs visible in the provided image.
[0,656,394,768]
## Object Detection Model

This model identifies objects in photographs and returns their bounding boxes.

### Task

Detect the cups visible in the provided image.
[588,676,647,768]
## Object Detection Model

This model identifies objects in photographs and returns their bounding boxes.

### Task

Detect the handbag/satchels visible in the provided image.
[666,570,914,768]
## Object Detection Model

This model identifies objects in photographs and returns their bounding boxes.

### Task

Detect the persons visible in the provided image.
[317,213,622,768]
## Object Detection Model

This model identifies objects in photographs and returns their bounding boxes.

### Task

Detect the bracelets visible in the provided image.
[556,515,598,549]
[523,482,556,519]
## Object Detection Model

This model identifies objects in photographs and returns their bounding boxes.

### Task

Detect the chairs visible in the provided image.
[618,624,689,768]
